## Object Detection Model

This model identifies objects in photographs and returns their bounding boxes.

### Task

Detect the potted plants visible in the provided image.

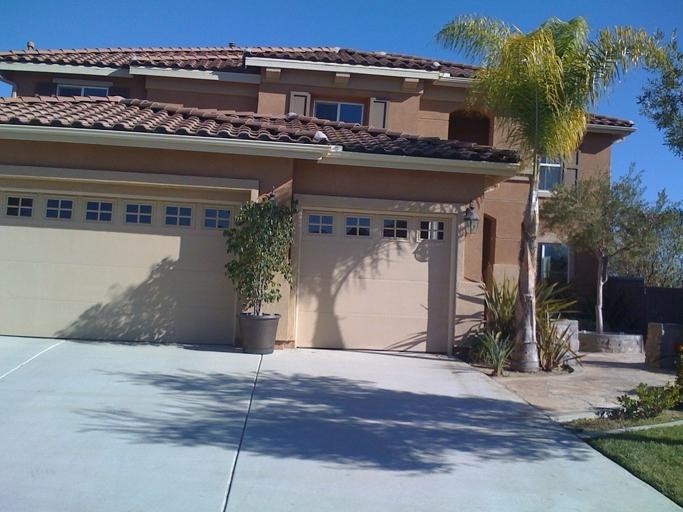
[224,196,297,355]
